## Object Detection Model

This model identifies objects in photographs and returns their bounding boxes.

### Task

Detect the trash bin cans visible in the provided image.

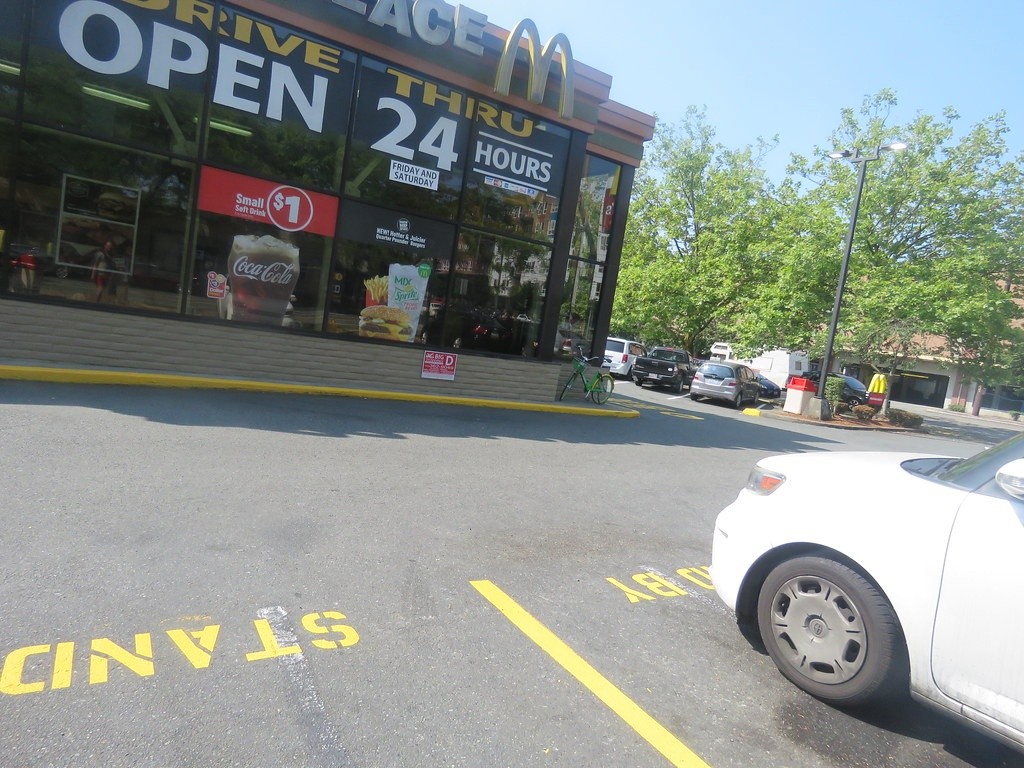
[783,376,818,415]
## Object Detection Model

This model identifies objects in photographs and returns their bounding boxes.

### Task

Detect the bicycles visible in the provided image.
[559,345,615,407]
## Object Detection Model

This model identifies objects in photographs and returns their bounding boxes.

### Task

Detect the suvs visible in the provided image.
[601,336,649,381]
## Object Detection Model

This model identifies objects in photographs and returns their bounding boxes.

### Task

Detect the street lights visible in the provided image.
[817,140,908,399]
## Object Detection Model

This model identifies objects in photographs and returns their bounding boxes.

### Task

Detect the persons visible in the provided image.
[89,240,118,305]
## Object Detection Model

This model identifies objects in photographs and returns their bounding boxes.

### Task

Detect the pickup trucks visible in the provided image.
[631,345,698,394]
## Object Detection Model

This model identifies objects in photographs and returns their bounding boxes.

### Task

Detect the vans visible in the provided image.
[709,342,755,362]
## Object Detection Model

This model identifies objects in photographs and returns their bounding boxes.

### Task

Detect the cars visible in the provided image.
[689,356,717,370]
[753,372,781,399]
[801,370,868,412]
[689,360,761,410]
[707,431,1024,757]
[1,224,231,303]
[411,298,594,360]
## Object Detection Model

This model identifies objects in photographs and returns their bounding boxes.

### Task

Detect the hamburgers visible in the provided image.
[357,305,412,341]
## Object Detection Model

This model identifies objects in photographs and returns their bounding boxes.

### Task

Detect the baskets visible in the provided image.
[570,356,591,375]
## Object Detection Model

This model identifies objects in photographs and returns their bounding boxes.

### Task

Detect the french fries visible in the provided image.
[364,274,389,298]
